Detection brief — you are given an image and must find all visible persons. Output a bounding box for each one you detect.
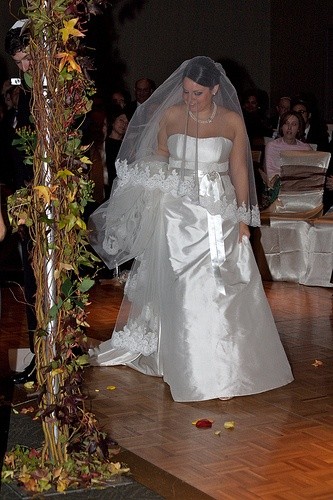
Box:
[241,92,333,214]
[0,33,94,385]
[86,56,296,403]
[0,78,155,192]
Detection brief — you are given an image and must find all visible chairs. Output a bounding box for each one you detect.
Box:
[261,136,333,289]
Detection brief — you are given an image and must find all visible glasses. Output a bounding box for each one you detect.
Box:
[291,99,304,104]
[298,110,310,114]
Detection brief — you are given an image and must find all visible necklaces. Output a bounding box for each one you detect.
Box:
[189,101,217,124]
